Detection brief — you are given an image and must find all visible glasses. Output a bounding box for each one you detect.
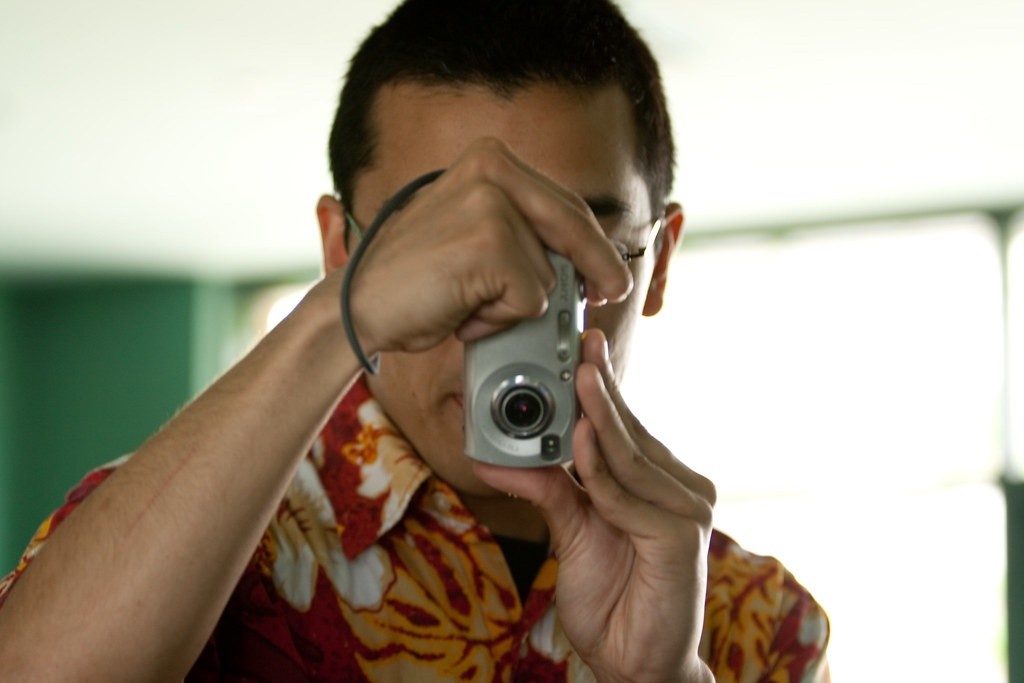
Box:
[340,208,664,265]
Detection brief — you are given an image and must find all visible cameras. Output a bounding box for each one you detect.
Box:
[464,254,589,471]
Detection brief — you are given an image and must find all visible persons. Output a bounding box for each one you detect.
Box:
[0,0,833,683]
